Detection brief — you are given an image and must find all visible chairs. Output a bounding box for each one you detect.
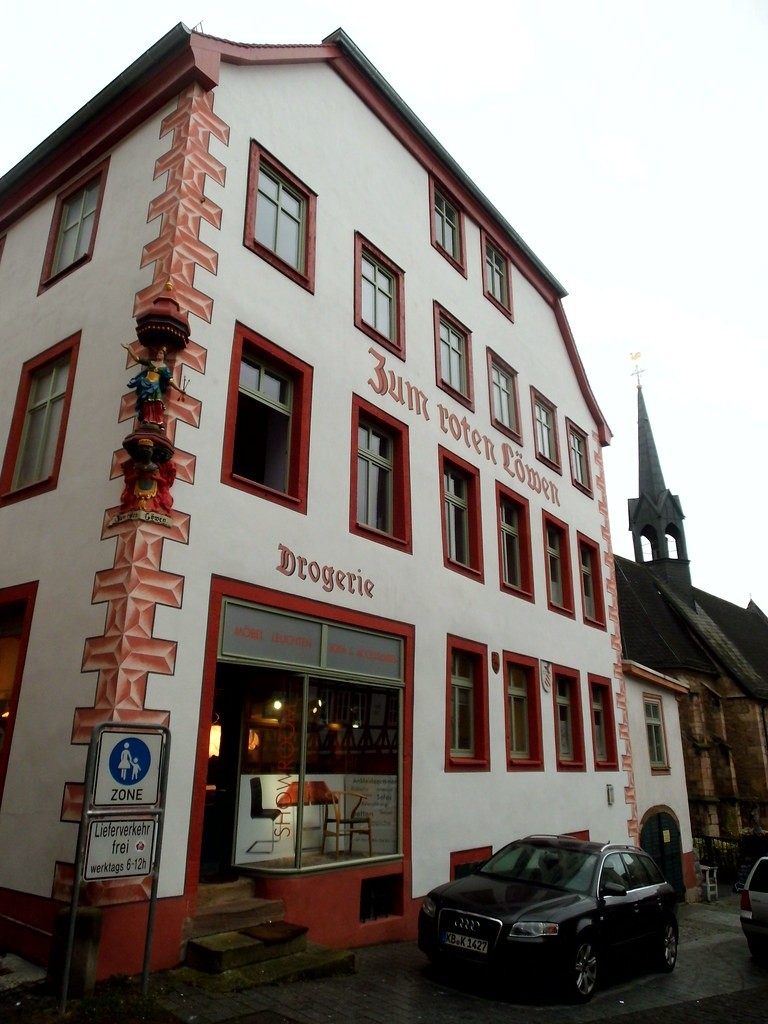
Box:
[321,790,371,861]
[529,852,560,885]
[245,777,281,853]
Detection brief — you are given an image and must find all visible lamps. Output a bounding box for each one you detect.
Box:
[606,784,615,806]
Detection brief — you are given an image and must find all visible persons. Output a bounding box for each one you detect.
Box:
[121,342,186,430]
[125,439,167,498]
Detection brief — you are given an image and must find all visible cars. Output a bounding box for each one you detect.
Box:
[739,854,768,962]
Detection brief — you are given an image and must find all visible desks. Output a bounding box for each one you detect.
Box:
[702,866,719,901]
[278,780,339,855]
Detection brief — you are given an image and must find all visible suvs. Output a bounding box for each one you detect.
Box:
[416,833,680,1005]
